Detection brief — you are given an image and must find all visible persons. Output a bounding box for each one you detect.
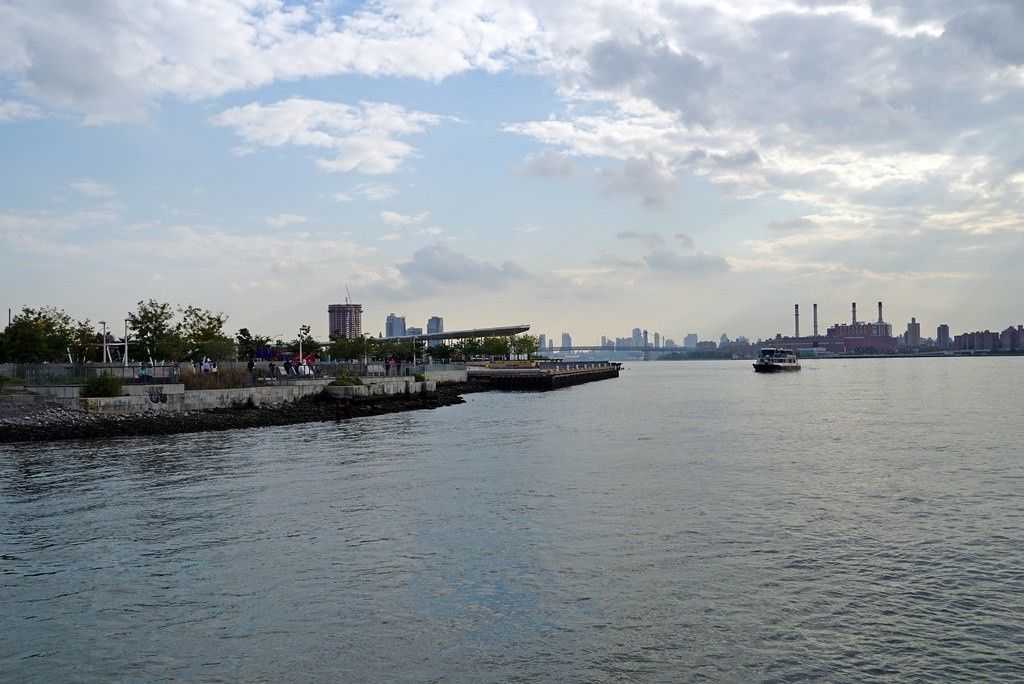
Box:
[385,355,401,376]
[138,366,148,378]
[247,359,319,379]
[203,360,217,373]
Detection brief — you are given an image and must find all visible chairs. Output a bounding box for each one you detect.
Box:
[368,364,386,377]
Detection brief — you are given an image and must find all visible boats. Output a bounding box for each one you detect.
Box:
[752,348,801,372]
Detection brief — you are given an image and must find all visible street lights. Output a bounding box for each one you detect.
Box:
[413,336,418,366]
[124,317,134,366]
[363,331,370,365]
[98,321,106,363]
[297,324,310,364]
